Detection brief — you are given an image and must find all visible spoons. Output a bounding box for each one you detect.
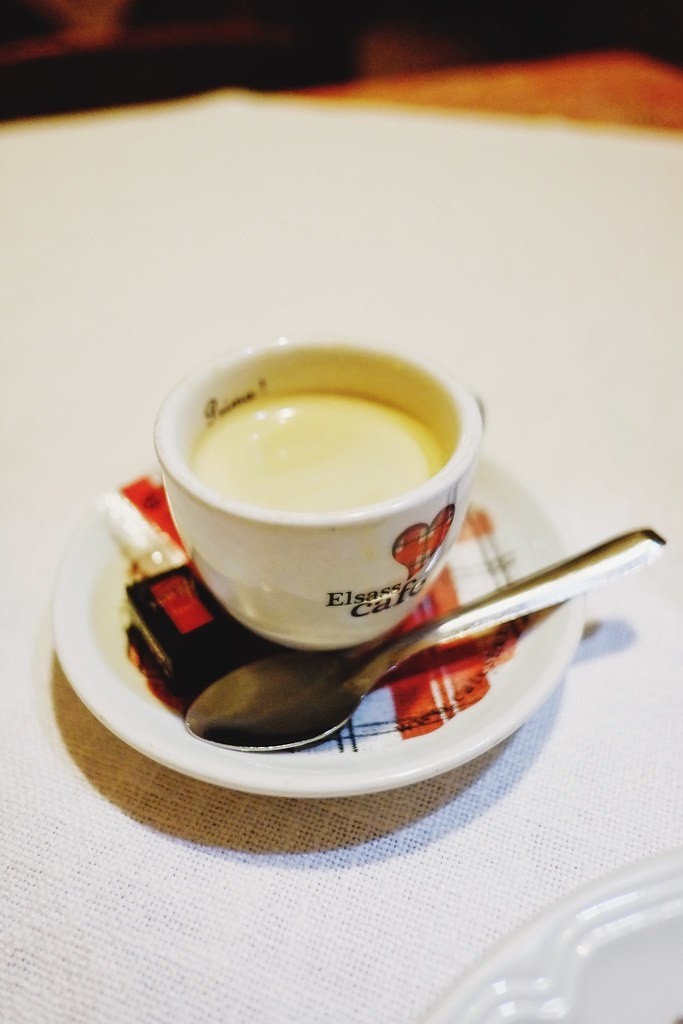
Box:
[184,527,665,746]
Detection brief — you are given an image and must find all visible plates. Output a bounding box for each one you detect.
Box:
[51,469,582,798]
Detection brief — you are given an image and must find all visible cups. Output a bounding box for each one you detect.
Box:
[152,337,484,650]
